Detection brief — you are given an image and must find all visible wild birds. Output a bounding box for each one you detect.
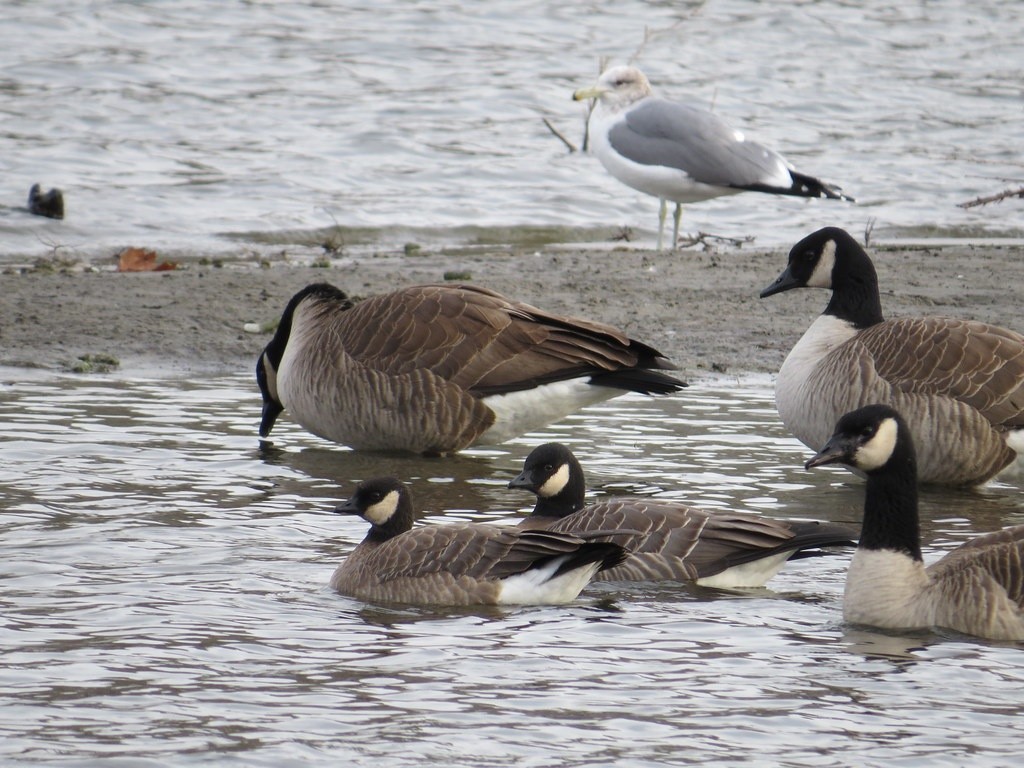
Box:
[255,283,689,455]
[805,403,1023,644]
[507,442,861,589]
[761,227,1024,492]
[572,66,855,252]
[328,476,651,607]
